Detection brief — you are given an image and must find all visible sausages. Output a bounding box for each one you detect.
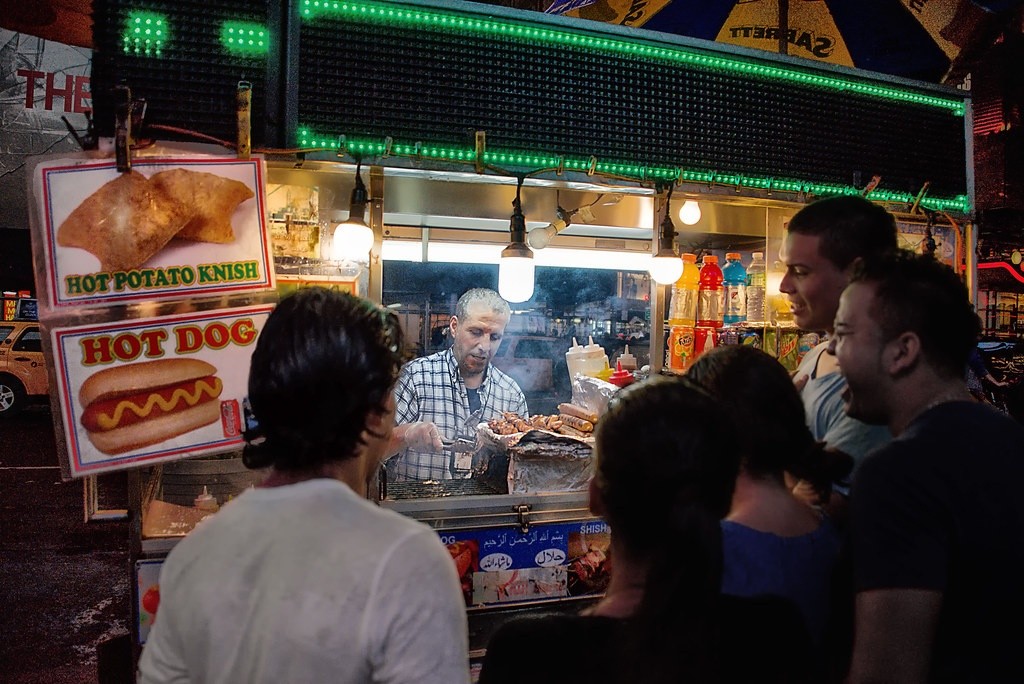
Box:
[488,404,599,437]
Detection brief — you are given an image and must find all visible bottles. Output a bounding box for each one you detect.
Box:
[597,361,615,382]
[616,344,638,373]
[583,335,610,380]
[565,337,585,386]
[194,486,219,513]
[720,253,748,323]
[696,256,724,327]
[667,253,700,327]
[609,361,634,387]
[745,252,766,324]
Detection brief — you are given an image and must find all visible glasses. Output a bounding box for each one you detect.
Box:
[608,374,717,414]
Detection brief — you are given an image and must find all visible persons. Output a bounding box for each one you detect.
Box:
[967,313,1009,402]
[138,286,472,684]
[826,247,1024,684]
[686,344,853,668]
[781,195,896,456]
[384,287,529,480]
[477,378,834,684]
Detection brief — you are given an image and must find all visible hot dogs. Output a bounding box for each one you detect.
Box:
[81,358,223,453]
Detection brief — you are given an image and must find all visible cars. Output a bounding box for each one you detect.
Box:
[614,326,645,341]
[488,336,560,392]
[0,321,56,413]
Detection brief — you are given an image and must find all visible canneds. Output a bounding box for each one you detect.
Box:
[671,324,820,374]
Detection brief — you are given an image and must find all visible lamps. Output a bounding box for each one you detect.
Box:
[332,162,375,259]
[498,178,535,304]
[529,190,606,249]
[649,182,684,286]
